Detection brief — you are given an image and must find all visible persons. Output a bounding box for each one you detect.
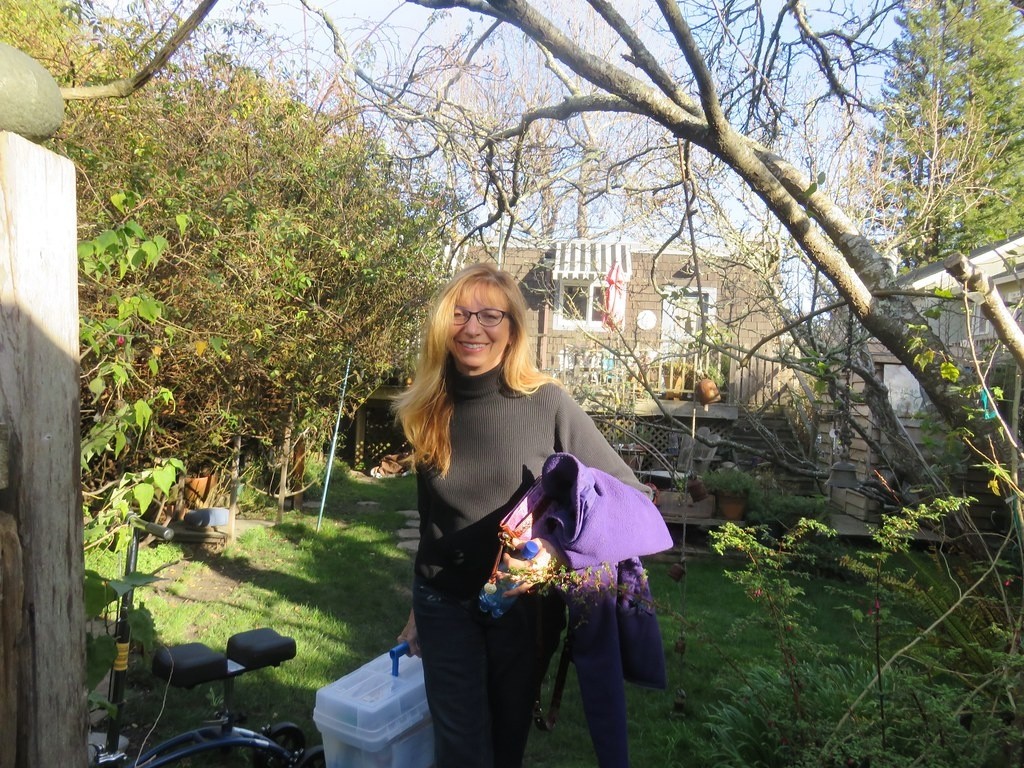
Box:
[391,263,652,767]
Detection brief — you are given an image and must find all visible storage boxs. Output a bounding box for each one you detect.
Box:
[312,641,436,768]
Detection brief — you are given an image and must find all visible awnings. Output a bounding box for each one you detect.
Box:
[550,241,632,282]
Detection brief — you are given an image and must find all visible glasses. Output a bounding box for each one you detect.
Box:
[453,307,516,327]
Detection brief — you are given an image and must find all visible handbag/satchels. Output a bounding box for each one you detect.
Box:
[485,474,577,595]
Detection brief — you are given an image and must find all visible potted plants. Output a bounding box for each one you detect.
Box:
[699,468,752,519]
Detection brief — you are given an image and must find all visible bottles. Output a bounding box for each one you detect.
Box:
[478,541,539,618]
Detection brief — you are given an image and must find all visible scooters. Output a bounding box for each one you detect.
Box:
[96,504,324,768]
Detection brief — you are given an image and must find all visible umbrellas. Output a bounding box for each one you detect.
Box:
[602,264,626,335]
